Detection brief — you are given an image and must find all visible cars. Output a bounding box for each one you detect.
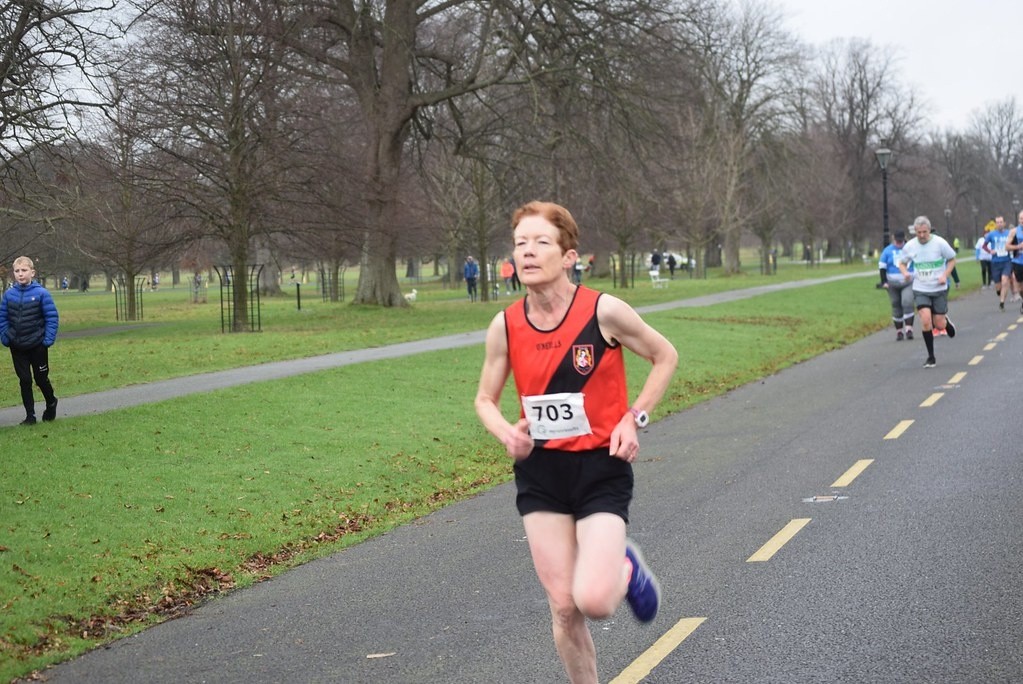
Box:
[646,253,696,270]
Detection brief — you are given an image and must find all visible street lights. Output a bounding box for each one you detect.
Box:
[1012,194,1019,226]
[875,136,891,247]
[972,207,979,243]
[944,205,951,243]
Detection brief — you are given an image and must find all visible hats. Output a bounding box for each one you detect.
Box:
[894,230,905,242]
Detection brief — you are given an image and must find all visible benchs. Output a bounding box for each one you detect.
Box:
[649,270,670,289]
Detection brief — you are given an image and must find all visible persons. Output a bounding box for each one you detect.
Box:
[288,267,295,285]
[574,255,594,282]
[501,254,521,295]
[0,256,59,425]
[667,253,675,276]
[143,273,158,292]
[474,200,678,684]
[195,271,200,292]
[974,210,1023,314]
[954,235,959,252]
[62,277,68,294]
[930,228,960,337]
[879,230,915,341]
[652,248,661,271]
[464,256,479,301]
[897,216,956,368]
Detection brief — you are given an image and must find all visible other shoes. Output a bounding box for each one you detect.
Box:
[1011,294,1022,303]
[997,290,1000,296]
[955,282,960,291]
[999,304,1005,312]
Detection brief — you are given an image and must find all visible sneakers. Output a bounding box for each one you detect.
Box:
[923,355,936,368]
[20,416,36,425]
[932,327,947,337]
[625,538,660,624]
[906,330,913,338]
[897,331,904,341]
[42,397,57,422]
[945,314,955,338]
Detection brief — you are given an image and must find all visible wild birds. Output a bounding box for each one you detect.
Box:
[405,289,417,302]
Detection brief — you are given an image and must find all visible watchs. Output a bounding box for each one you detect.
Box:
[628,408,650,427]
